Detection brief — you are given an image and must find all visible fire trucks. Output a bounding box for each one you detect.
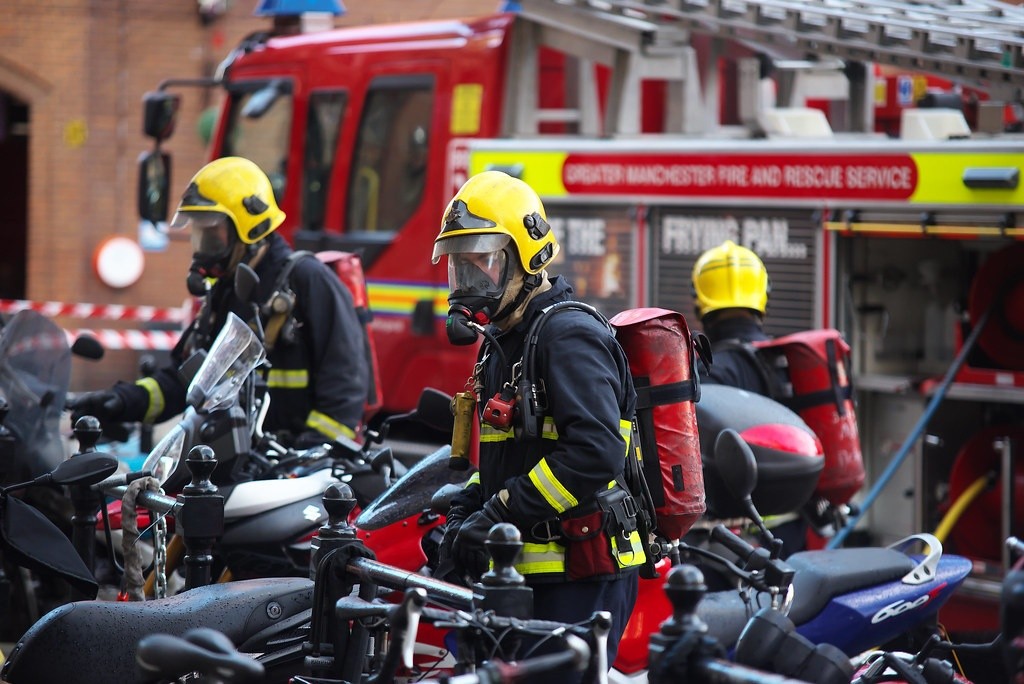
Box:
[136,0,1024,684]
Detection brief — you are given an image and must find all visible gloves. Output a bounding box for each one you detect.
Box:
[71,378,148,443]
[432,494,511,587]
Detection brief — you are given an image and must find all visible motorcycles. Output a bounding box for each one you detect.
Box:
[0,307,1024,684]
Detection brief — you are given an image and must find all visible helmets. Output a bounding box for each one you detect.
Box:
[177,157,286,243]
[691,239,767,321]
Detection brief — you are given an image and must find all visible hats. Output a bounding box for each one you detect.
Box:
[435,171,560,275]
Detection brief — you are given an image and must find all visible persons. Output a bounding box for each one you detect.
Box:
[69,156,372,454]
[692,240,794,409]
[431,169,651,684]
